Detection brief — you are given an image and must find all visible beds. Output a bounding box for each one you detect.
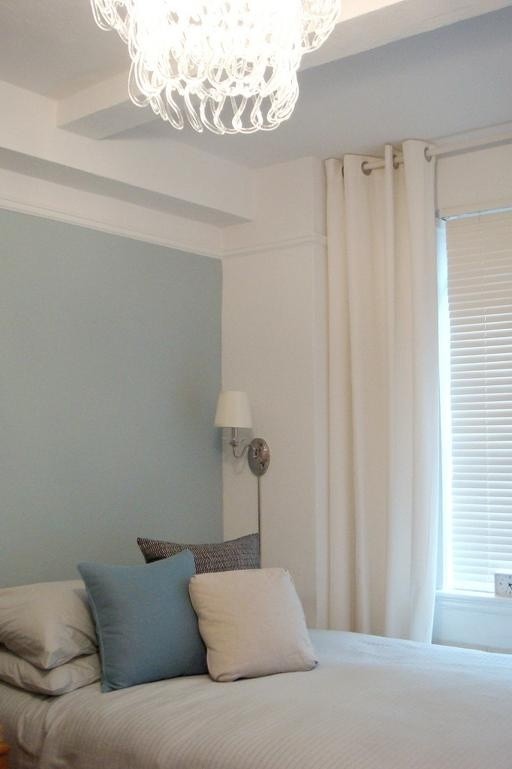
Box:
[1,630,512,769]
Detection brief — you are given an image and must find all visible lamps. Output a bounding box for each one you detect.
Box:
[88,1,343,134]
[214,390,268,479]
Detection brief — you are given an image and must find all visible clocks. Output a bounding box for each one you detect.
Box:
[495,573,512,597]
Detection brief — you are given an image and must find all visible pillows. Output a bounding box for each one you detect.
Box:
[73,549,208,694]
[190,566,318,684]
[2,578,101,670]
[1,645,101,697]
[137,534,263,573]
